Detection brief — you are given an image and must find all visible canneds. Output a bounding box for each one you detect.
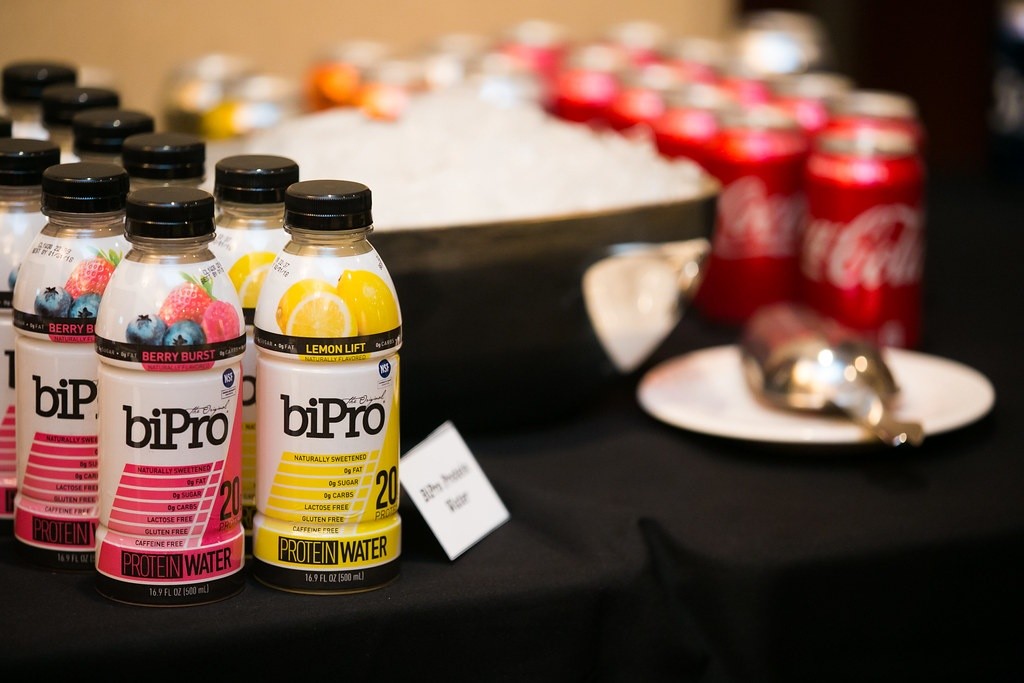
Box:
[304,19,929,347]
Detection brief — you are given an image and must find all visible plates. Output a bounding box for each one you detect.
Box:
[637,345,994,443]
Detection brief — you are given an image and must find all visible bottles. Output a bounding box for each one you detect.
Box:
[0,136,60,540]
[12,163,132,563]
[211,154,301,547]
[0,59,209,193]
[94,188,247,607]
[249,181,404,595]
